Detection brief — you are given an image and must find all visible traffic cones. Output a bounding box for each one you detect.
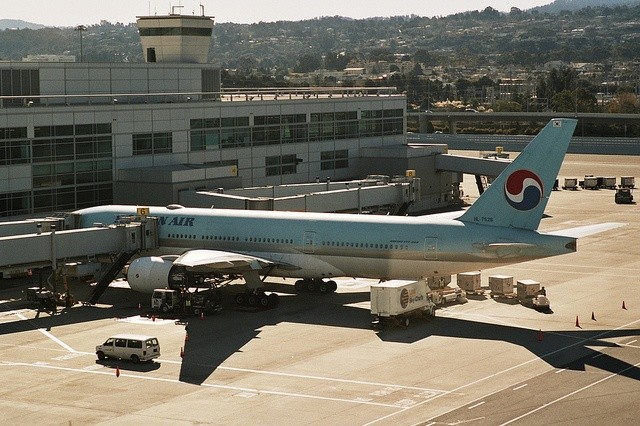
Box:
[184,331,189,342]
[576,316,579,326]
[198,309,205,321]
[592,312,594,319]
[622,300,625,309]
[116,367,119,377]
[537,329,544,341]
[180,347,184,357]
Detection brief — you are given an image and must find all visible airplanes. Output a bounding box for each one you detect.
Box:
[71,117,578,308]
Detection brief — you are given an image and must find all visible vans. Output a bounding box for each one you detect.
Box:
[95,335,161,364]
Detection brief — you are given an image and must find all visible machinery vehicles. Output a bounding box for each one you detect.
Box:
[614,189,634,204]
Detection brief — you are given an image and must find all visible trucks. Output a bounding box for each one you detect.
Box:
[427,272,551,312]
[150,286,214,316]
[369,279,437,327]
